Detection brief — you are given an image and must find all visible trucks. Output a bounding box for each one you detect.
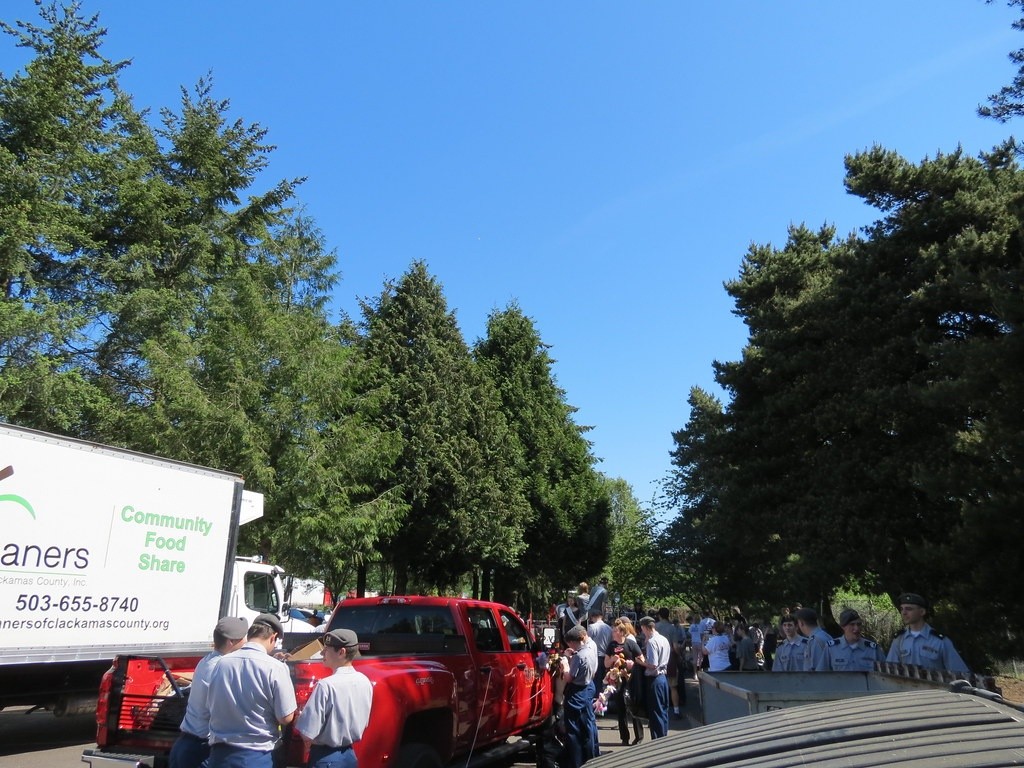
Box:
[0,419,317,718]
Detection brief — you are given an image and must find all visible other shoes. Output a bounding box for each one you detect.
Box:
[674,712,683,720]
[622,736,643,746]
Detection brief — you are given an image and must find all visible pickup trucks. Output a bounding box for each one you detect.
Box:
[84,592,557,768]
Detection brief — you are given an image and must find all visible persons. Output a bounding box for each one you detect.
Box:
[796,606,835,672]
[634,615,671,741]
[167,615,249,768]
[884,591,971,674]
[293,628,375,768]
[603,617,646,747]
[550,628,596,768]
[301,573,804,671]
[563,625,600,759]
[200,613,299,768]
[585,608,614,717]
[772,614,811,672]
[652,607,684,721]
[813,608,887,671]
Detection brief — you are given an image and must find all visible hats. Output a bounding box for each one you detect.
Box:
[620,616,630,623]
[796,607,817,622]
[322,629,359,649]
[779,614,798,625]
[839,610,861,625]
[254,612,286,640]
[897,593,927,607]
[562,628,581,640]
[657,607,669,619]
[638,616,654,625]
[588,609,603,617]
[216,614,250,641]
[570,624,586,633]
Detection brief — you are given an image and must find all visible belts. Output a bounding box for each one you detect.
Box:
[178,730,209,743]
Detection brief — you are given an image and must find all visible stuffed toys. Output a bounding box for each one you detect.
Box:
[592,653,631,717]
